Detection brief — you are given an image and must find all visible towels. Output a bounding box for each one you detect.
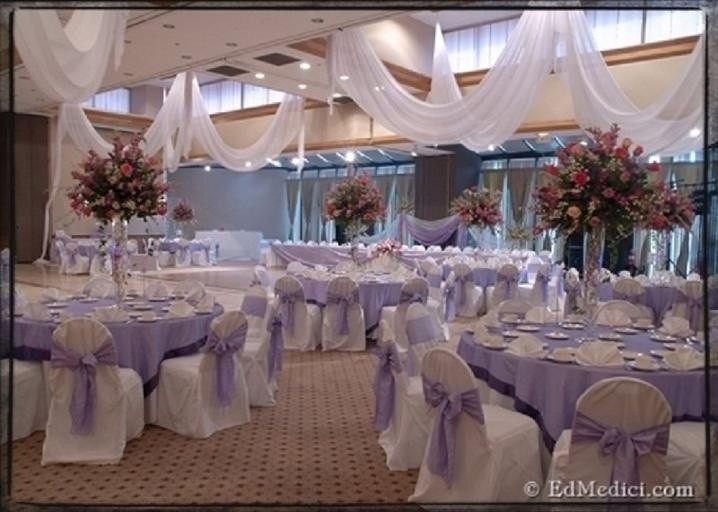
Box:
[168,302,194,318]
[576,342,625,368]
[525,307,555,323]
[482,310,502,328]
[37,288,67,302]
[663,318,691,337]
[665,348,701,371]
[89,283,115,299]
[184,289,203,302]
[24,303,53,323]
[196,294,215,310]
[507,336,548,358]
[599,311,632,328]
[92,308,129,323]
[473,323,489,346]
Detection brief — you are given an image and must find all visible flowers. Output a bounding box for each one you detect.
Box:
[452,186,504,224]
[62,131,170,221]
[174,198,191,220]
[323,172,388,223]
[533,124,696,236]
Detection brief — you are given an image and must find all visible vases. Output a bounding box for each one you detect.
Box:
[350,216,360,260]
[583,228,606,291]
[111,216,131,280]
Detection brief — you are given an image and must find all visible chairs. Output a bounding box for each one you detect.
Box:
[671,421,708,500]
[158,310,251,440]
[39,318,144,460]
[521,264,552,301]
[668,280,704,332]
[375,319,426,472]
[544,377,672,501]
[404,302,438,373]
[273,276,321,351]
[241,299,285,408]
[429,271,456,342]
[454,264,485,317]
[613,279,646,306]
[138,236,220,267]
[267,237,378,261]
[492,264,521,303]
[393,228,544,265]
[410,348,552,503]
[378,277,429,343]
[49,230,107,275]
[320,276,367,351]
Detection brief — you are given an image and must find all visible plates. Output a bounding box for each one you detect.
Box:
[632,323,655,329]
[599,334,623,339]
[467,326,488,333]
[483,321,500,328]
[650,335,676,341]
[610,342,624,347]
[622,351,639,359]
[614,327,638,333]
[628,360,660,371]
[14,291,214,322]
[548,353,573,362]
[650,349,673,357]
[482,341,508,348]
[564,324,583,329]
[545,332,570,339]
[664,343,684,350]
[516,326,539,331]
[502,331,519,337]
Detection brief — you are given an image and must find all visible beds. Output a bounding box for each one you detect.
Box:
[13,290,223,384]
